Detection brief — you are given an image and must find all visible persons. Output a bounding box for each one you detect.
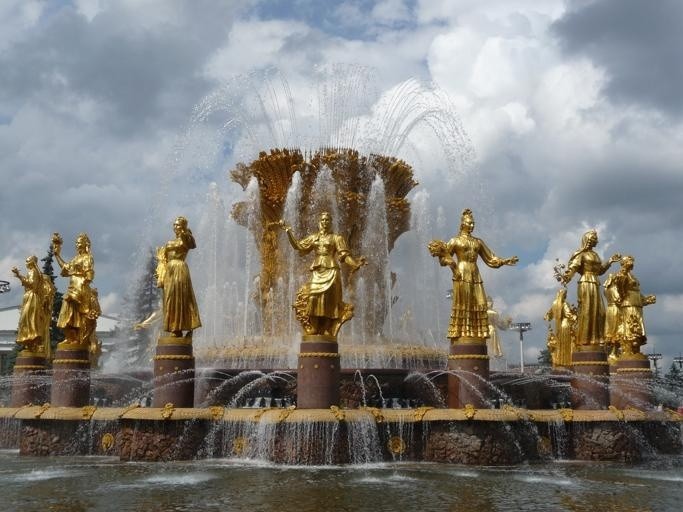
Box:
[49,231,95,346]
[10,254,47,353]
[275,211,369,336]
[543,289,578,367]
[88,286,102,354]
[484,296,514,358]
[553,228,624,347]
[603,273,623,346]
[428,209,519,339]
[151,216,202,338]
[617,252,657,352]
[39,273,54,359]
[132,300,165,348]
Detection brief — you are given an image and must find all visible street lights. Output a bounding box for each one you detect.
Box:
[0,280,10,294]
[648,353,663,379]
[508,323,531,375]
[672,357,683,371]
